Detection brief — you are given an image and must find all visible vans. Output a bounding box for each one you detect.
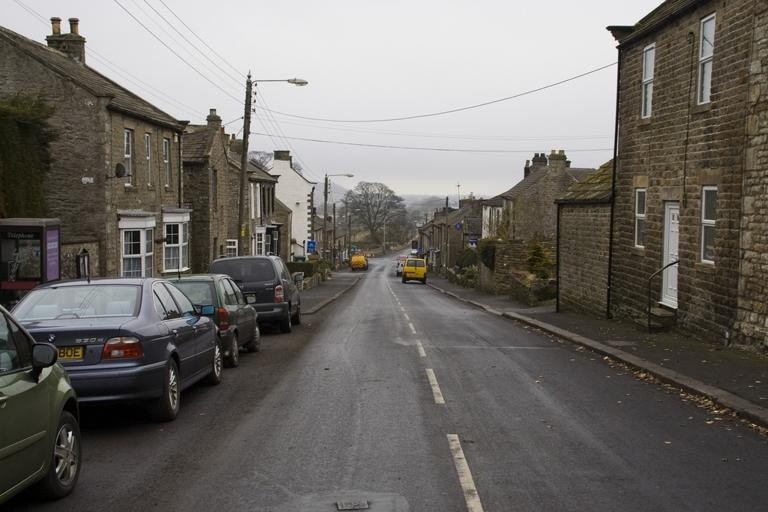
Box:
[402,258,427,284]
[352,256,368,270]
[209,255,299,335]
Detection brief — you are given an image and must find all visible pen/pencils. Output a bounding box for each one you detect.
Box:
[402,258,427,284]
[352,256,368,270]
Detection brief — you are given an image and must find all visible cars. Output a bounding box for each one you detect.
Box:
[0,304,83,505]
[8,278,224,422]
[162,273,260,368]
[396,262,403,276]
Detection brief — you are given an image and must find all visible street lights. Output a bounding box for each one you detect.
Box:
[323,173,354,261]
[349,208,360,265]
[238,69,308,256]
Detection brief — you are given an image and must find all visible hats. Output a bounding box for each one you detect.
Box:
[396,262,403,276]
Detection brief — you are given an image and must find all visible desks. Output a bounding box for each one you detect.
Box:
[238,69,308,256]
[323,173,354,261]
[349,208,360,265]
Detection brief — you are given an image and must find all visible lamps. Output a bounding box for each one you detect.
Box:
[31,301,130,318]
